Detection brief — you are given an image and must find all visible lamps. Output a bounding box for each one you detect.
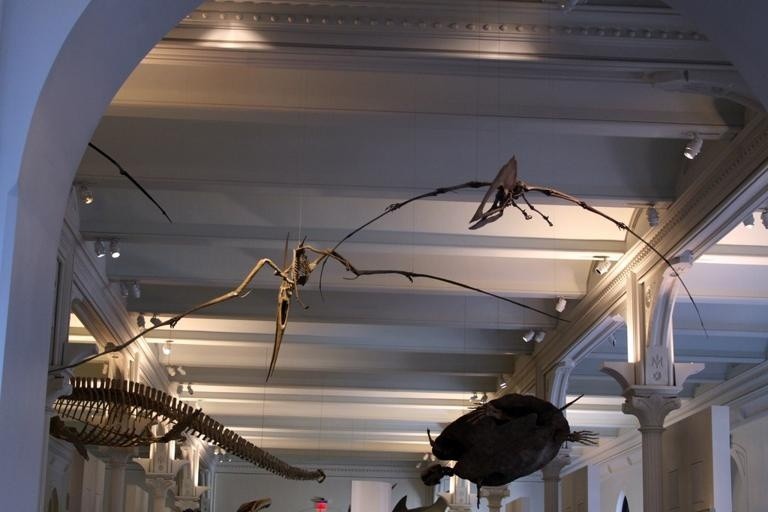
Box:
[645,204,662,229]
[593,256,612,276]
[682,135,704,162]
[469,371,512,403]
[555,296,567,315]
[522,329,546,344]
[75,182,194,396]
